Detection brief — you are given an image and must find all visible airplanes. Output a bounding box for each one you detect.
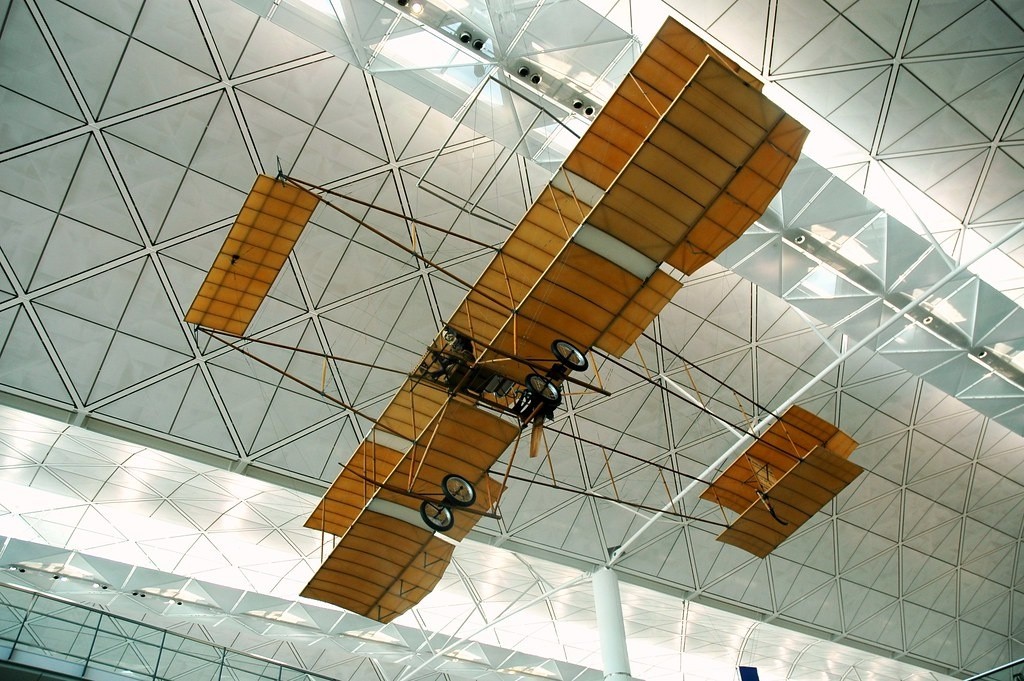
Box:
[181,14,866,625]
